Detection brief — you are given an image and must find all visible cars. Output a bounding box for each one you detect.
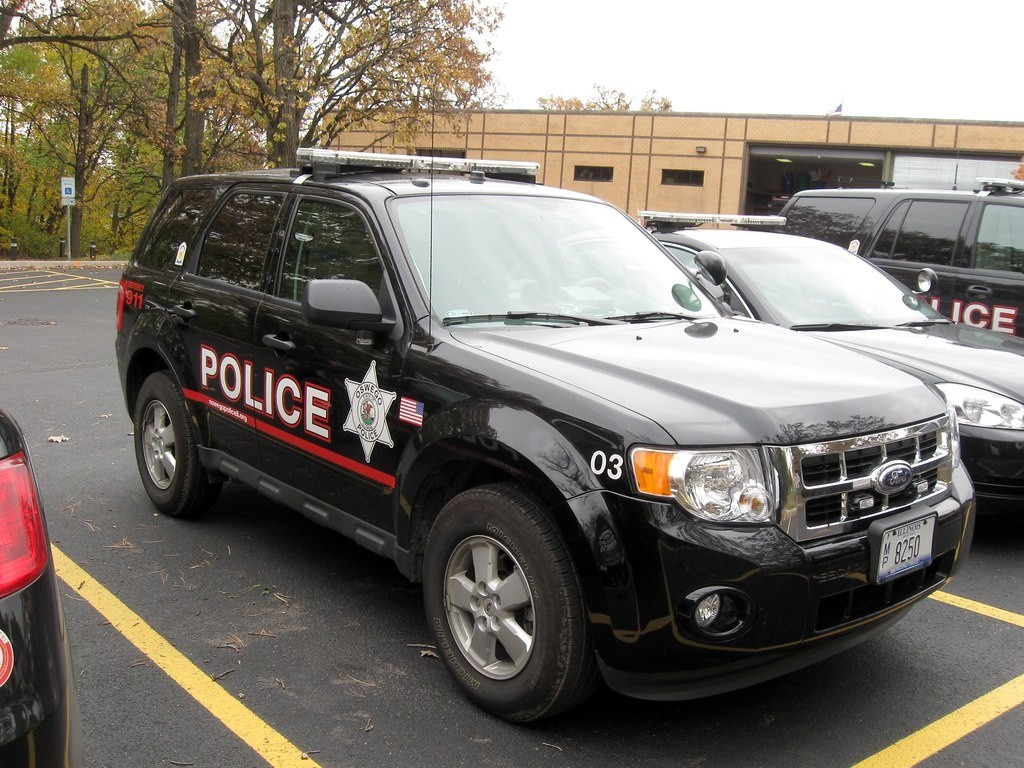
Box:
[556,207,1024,526]
[0,411,72,768]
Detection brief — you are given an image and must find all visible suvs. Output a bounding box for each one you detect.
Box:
[775,175,1023,343]
[115,149,976,726]
